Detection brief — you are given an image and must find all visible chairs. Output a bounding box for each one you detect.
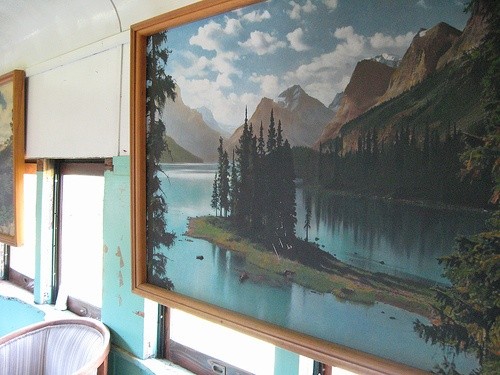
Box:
[0,318,112,375]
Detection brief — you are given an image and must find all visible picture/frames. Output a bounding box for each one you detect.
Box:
[0,69,26,247]
[129,0,500,375]
